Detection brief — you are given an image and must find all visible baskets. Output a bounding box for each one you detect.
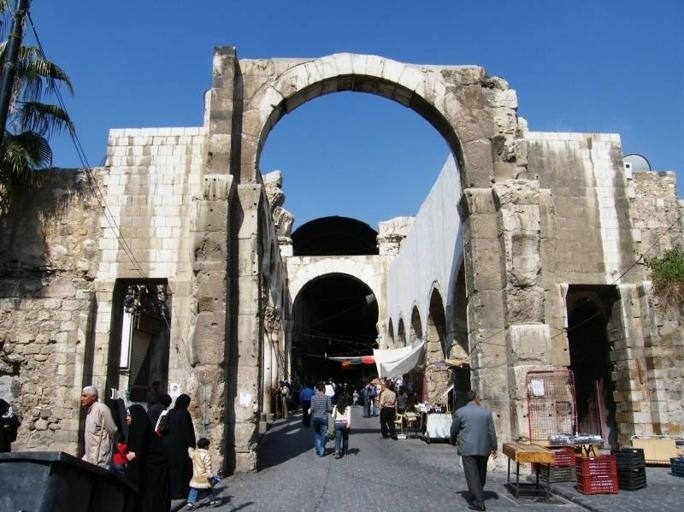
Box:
[539,446,578,467]
[609,446,647,469]
[669,456,684,477]
[575,454,617,477]
[575,475,618,494]
[616,467,646,490]
[538,464,577,483]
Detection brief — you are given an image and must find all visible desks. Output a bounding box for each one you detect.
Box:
[502,442,555,500]
[532,440,605,457]
[421,412,453,445]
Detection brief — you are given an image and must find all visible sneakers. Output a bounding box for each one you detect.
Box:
[314,446,350,460]
[186,499,224,510]
[467,502,485,511]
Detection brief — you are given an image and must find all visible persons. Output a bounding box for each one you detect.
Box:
[80,380,222,511]
[278,373,420,459]
[449,390,498,511]
